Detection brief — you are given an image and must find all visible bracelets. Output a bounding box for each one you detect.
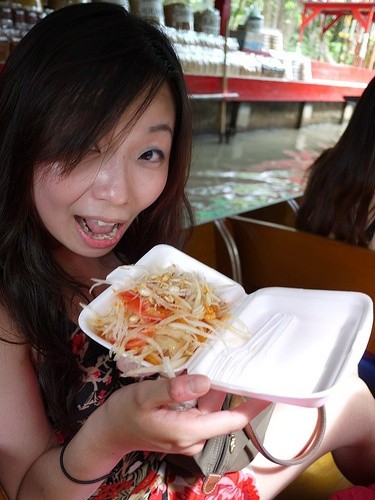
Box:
[59,441,124,484]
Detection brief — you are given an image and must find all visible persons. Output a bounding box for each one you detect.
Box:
[295,72,374,255]
[0,2,375,500]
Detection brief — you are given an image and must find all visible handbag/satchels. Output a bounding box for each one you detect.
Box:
[153,371,327,481]
